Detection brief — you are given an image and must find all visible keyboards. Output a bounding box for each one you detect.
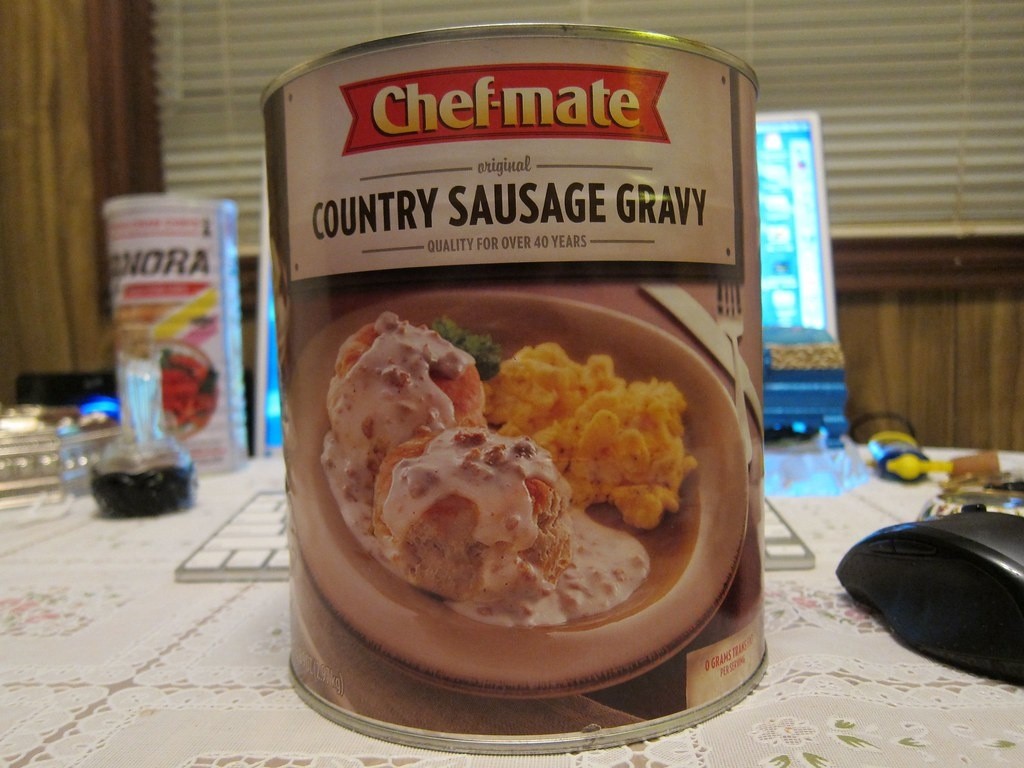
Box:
[172,488,813,586]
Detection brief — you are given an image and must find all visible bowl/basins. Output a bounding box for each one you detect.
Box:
[285,288,751,701]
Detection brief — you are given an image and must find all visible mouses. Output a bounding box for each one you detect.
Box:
[836,501,1022,688]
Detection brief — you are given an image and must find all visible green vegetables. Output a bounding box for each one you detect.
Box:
[429,315,501,381]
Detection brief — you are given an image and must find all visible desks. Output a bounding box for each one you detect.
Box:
[0,439,1024,768]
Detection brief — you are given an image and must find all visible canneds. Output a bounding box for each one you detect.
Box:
[103,193,248,473]
[257,17,769,756]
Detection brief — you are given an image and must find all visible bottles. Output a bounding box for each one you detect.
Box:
[87,345,195,519]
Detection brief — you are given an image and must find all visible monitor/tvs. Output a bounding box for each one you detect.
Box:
[755,112,851,440]
